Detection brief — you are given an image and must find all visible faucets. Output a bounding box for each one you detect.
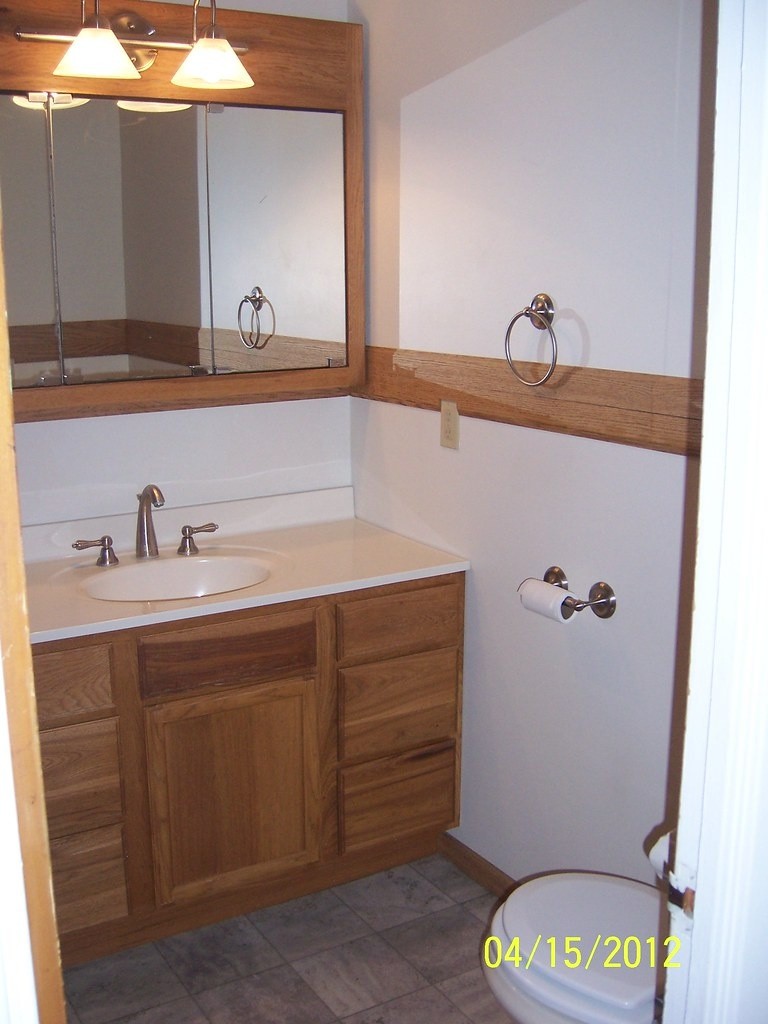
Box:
[136,484,165,559]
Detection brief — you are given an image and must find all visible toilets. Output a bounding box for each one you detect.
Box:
[479,829,676,1024]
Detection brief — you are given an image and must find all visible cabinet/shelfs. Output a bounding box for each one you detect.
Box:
[30,570,466,968]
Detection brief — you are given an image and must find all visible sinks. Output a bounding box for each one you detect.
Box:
[83,557,270,600]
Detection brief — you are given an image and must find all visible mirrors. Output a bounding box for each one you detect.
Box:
[0,87,347,390]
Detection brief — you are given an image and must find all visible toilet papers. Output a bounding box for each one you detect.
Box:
[516,577,578,624]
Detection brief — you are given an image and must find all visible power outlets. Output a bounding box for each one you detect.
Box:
[440,398,459,450]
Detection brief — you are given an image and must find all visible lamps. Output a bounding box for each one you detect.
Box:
[16,1,257,90]
